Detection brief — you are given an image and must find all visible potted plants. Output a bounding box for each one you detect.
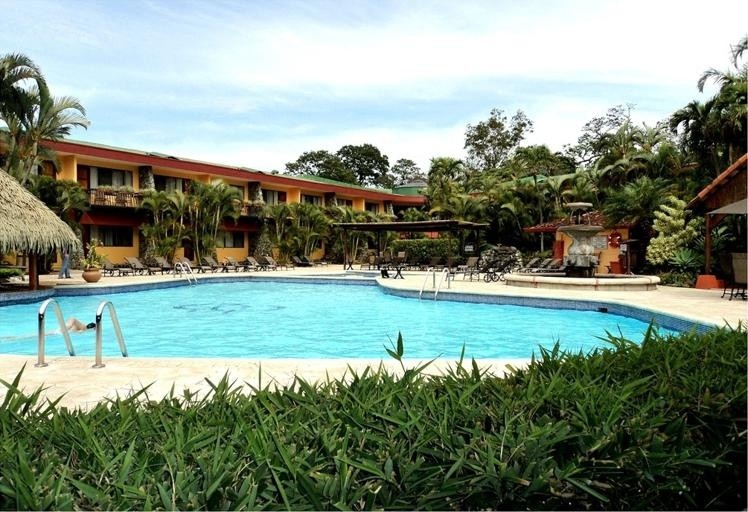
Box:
[81,241,102,282]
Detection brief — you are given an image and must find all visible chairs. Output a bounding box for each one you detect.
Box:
[377,257,563,282]
[102,255,294,277]
[722,264,744,302]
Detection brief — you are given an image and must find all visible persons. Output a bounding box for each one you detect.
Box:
[57,244,73,279]
